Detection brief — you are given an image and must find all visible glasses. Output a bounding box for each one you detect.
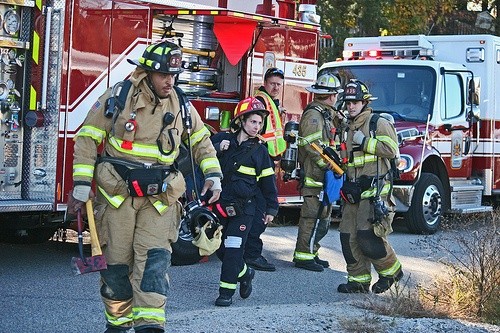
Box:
[270,68,284,75]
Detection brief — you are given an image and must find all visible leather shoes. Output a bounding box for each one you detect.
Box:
[246,256,275,271]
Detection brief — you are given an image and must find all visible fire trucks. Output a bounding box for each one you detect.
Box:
[0,0,332,265]
[317,35,500,235]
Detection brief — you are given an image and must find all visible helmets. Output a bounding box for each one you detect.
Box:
[230,97,270,127]
[339,79,378,101]
[305,72,344,94]
[127,40,184,74]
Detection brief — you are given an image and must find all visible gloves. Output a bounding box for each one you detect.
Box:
[353,131,365,145]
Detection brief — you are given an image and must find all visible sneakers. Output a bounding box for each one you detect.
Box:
[314,256,328,268]
[295,260,324,271]
[240,268,255,298]
[372,271,403,293]
[338,282,370,293]
[215,293,233,306]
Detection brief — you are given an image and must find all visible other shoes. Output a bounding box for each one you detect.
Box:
[104,328,131,333]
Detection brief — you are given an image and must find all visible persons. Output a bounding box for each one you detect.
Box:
[244,68,286,272]
[292,73,344,271]
[71,43,224,333]
[325,79,404,294]
[211,97,279,307]
